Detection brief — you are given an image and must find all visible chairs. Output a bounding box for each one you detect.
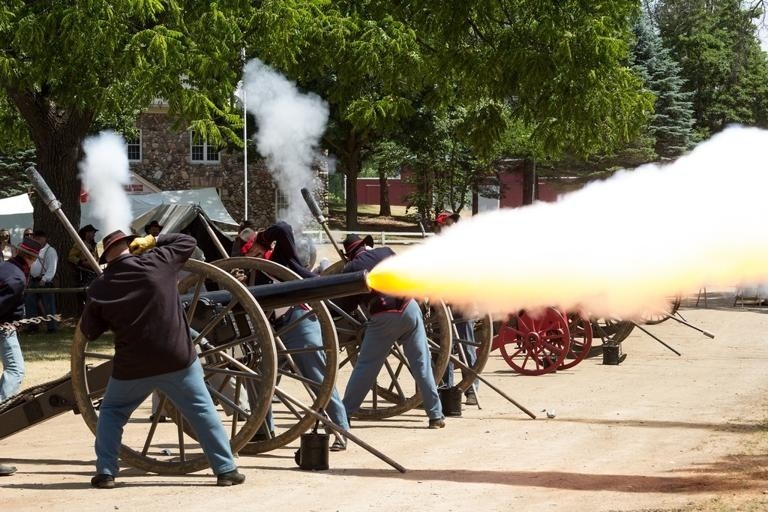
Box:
[150,396,282,442]
[217,470,246,487]
[330,434,347,452]
[91,474,114,489]
[429,419,445,430]
[0,462,18,476]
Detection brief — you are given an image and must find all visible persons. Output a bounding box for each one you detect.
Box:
[330,233,444,429]
[433,212,479,404]
[231,220,350,452]
[145,220,164,236]
[0,224,101,333]
[0,237,44,475]
[80,231,245,488]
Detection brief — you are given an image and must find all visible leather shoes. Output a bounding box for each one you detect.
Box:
[129,235,156,257]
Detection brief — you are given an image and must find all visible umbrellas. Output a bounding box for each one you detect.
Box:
[466,395,477,406]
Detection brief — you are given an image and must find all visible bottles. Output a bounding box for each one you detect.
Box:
[144,220,164,236]
[435,210,461,227]
[239,227,266,254]
[99,229,140,264]
[341,234,375,256]
[13,239,42,262]
[77,224,99,237]
